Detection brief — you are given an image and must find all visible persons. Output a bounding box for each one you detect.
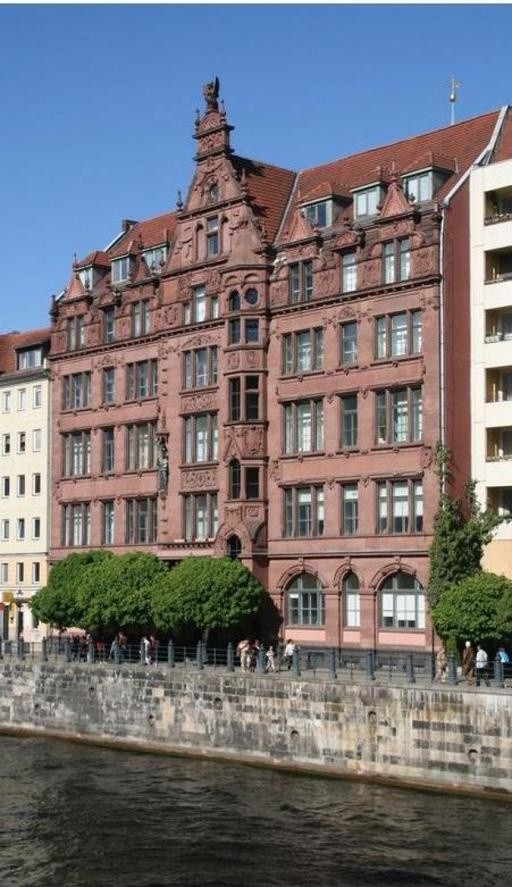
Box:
[495,648,510,683]
[237,637,252,671]
[250,637,261,672]
[265,644,275,671]
[433,647,448,683]
[474,644,489,686]
[282,638,299,669]
[462,639,475,686]
[66,630,157,663]
